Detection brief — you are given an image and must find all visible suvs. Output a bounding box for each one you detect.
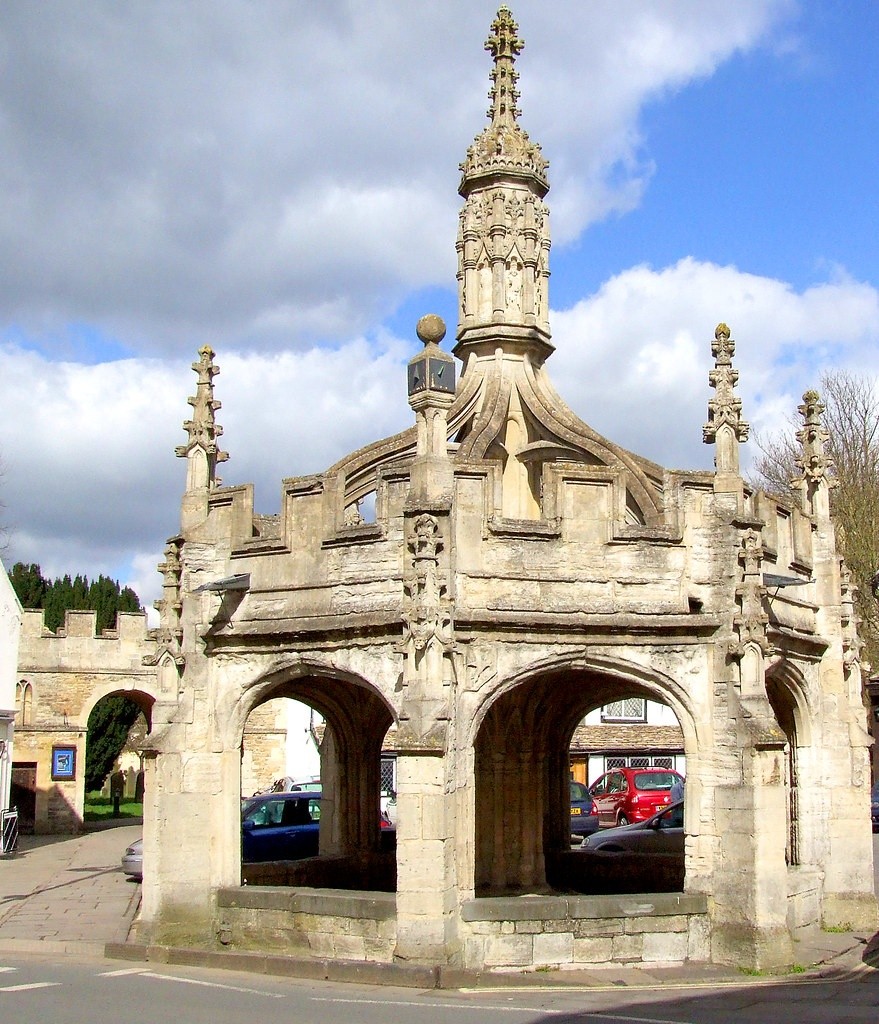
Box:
[589,766,686,829]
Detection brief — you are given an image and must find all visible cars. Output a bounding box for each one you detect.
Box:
[242,780,397,863]
[122,838,142,877]
[569,781,599,837]
[871,780,879,834]
[580,800,684,853]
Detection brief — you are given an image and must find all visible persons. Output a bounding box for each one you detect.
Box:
[670,777,686,814]
[623,778,627,787]
[386,791,397,824]
